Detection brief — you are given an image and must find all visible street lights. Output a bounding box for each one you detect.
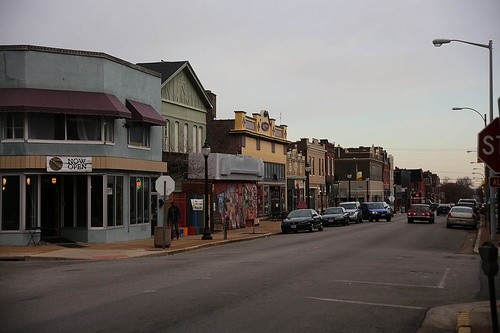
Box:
[305,163,311,208]
[392,183,397,213]
[451,106,487,129]
[432,39,495,240]
[201,142,213,240]
[365,176,369,202]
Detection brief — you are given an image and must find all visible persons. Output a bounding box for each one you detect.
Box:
[167,201,181,240]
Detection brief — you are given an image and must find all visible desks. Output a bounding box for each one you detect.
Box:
[27,226,50,248]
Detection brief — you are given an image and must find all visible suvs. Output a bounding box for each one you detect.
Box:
[338,200,363,223]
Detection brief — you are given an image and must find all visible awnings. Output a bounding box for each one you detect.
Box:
[0,87,131,119]
[125,99,167,126]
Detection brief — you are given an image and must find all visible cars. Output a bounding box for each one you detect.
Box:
[281,209,323,232]
[361,201,393,222]
[446,198,482,229]
[322,206,350,226]
[431,203,456,216]
[407,203,434,224]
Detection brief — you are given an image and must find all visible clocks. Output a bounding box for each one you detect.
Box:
[262,122,269,131]
